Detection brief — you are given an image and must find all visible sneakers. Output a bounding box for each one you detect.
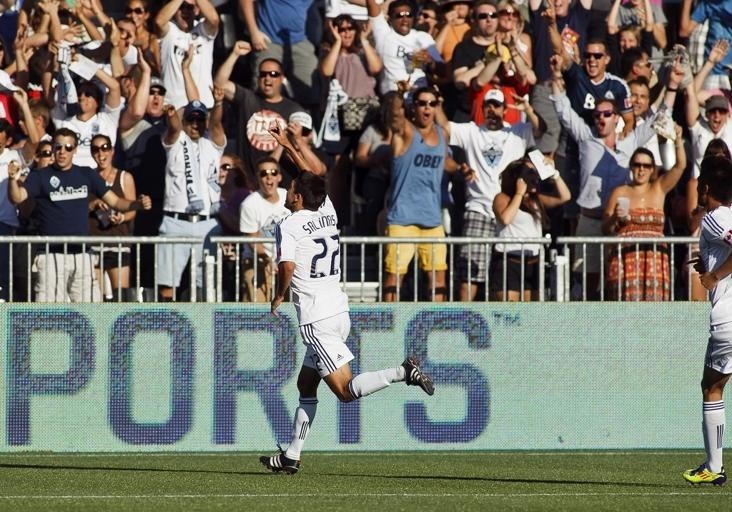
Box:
[402,357,434,395]
[683,463,726,487]
[260,443,299,474]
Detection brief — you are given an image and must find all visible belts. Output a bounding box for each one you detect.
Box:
[509,257,538,265]
[165,211,221,221]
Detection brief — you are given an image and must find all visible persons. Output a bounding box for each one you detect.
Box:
[1,2,732,299]
[259,119,434,473]
[681,153,732,488]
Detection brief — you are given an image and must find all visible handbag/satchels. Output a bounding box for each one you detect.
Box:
[343,95,379,129]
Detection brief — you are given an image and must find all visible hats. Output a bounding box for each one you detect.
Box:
[704,95,729,114]
[484,90,504,102]
[184,101,206,114]
[150,77,165,86]
[289,112,312,130]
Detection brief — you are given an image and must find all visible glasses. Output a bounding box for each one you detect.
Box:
[261,169,278,177]
[339,26,355,33]
[418,100,437,108]
[640,62,652,68]
[633,162,653,170]
[393,11,412,19]
[418,11,436,21]
[257,70,279,78]
[91,144,109,153]
[593,111,613,118]
[222,164,231,169]
[126,7,143,15]
[54,143,72,151]
[478,13,498,19]
[583,52,602,59]
[500,10,518,18]
[150,89,166,95]
[43,150,52,156]
[184,114,203,123]
